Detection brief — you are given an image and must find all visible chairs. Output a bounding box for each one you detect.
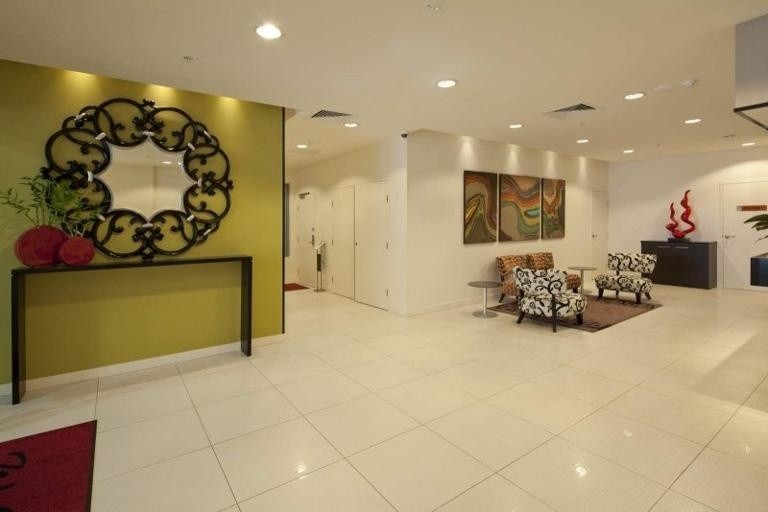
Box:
[495,255,530,303]
[529,252,582,293]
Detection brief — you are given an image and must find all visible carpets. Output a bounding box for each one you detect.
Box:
[284,281,310,292]
[486,293,664,334]
[0,417,99,512]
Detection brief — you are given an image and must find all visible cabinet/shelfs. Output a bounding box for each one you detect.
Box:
[640,240,718,290]
[750,251,768,287]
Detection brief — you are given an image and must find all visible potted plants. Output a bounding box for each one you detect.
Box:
[1,171,81,269]
[49,193,108,267]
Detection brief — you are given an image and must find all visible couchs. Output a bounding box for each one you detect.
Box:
[594,252,658,305]
[511,266,588,333]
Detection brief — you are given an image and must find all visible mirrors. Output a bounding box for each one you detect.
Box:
[35,96,236,264]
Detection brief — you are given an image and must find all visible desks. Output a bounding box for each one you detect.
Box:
[11,253,255,404]
[568,265,599,293]
[467,280,504,320]
